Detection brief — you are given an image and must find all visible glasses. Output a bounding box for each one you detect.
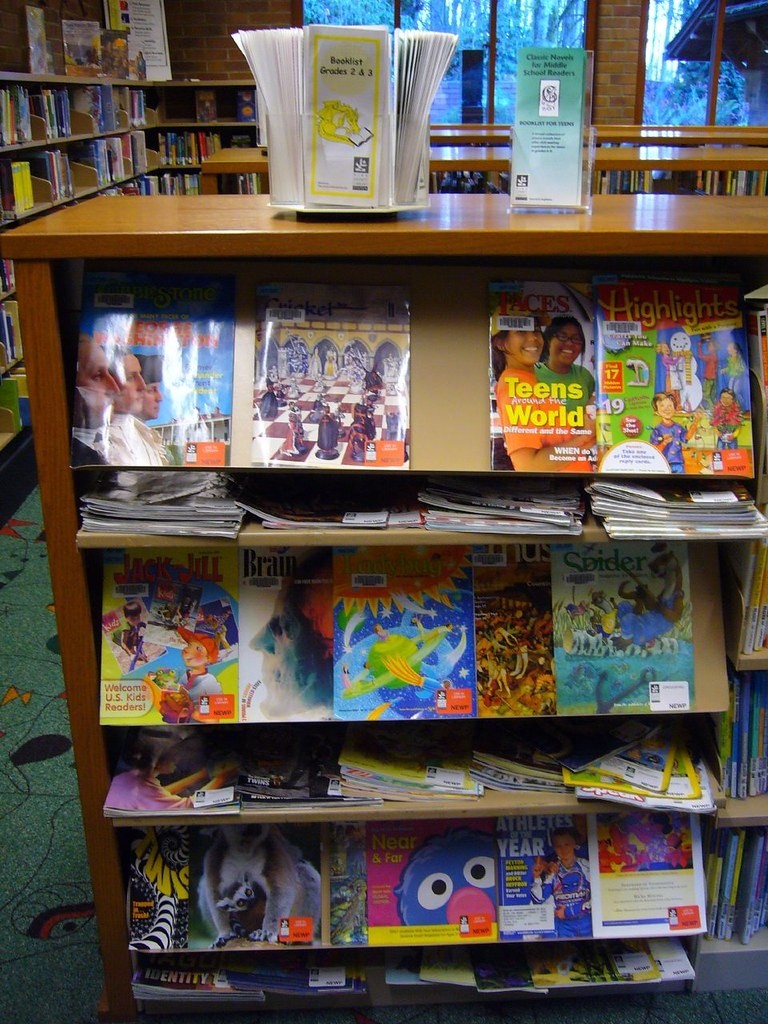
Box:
[548,332,585,345]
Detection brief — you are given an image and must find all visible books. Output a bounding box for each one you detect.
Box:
[488,269,755,479]
[704,303,768,945]
[0,259,32,432]
[248,284,410,470]
[79,476,768,1002]
[69,258,238,470]
[0,5,261,220]
[597,169,767,196]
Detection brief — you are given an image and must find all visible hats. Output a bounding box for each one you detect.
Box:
[134,354,163,384]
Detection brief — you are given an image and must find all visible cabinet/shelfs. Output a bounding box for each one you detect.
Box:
[0,71,768,1024]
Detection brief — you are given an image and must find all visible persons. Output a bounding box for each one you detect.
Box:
[528,814,593,937]
[495,316,596,472]
[74,334,170,467]
